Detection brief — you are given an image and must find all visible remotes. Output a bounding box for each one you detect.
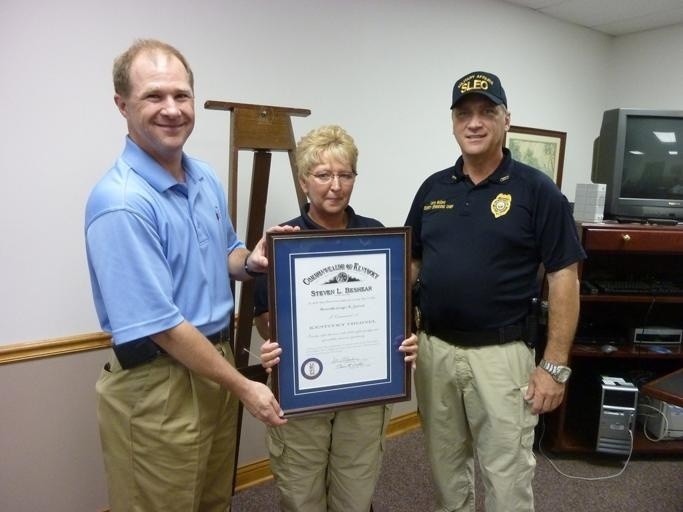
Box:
[647,218,678,225]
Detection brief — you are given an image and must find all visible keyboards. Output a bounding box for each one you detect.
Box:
[597,280,683,296]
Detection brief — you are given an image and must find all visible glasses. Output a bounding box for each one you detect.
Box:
[307,169,355,185]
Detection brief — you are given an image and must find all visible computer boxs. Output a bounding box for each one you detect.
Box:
[594,374,639,456]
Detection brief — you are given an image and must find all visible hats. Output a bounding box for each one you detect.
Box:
[449,70,508,112]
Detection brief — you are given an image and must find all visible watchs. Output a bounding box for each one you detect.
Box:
[539,357,574,385]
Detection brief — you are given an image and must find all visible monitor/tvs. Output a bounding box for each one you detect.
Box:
[591,108,683,222]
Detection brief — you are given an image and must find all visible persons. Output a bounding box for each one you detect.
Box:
[84,35,302,512]
[402,70,588,511]
[252,124,420,511]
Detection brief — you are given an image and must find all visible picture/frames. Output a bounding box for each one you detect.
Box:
[502,125,567,191]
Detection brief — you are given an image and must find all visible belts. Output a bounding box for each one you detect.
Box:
[419,319,524,349]
[204,324,230,346]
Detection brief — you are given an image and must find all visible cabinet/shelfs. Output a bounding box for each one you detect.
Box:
[534,222,683,453]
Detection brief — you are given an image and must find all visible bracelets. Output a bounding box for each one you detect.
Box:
[243,250,265,278]
